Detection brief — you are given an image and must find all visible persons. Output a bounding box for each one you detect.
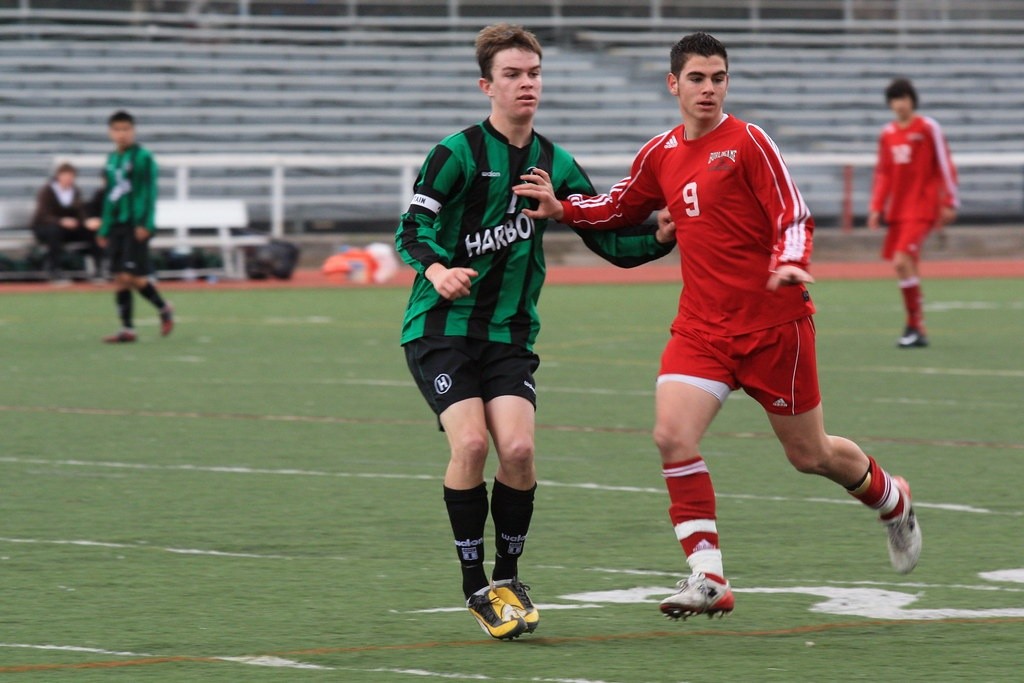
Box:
[32,164,111,278]
[96,111,175,342]
[868,79,960,346]
[510,34,922,618]
[395,23,677,639]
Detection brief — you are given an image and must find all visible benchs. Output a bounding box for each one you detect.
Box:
[2,195,269,281]
[0,0,1024,229]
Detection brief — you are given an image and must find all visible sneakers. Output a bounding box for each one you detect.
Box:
[659,571,735,618]
[465,583,530,641]
[160,303,173,335]
[877,474,923,575]
[103,329,137,341]
[899,329,927,346]
[489,571,541,632]
